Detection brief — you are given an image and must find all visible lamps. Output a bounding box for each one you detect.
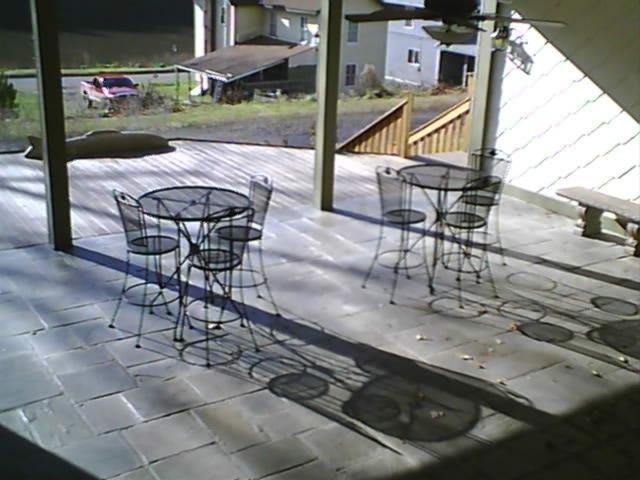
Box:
[422,19,479,47]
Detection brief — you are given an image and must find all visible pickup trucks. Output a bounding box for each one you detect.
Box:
[80,74,144,109]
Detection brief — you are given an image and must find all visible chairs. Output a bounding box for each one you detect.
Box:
[108,173,284,374]
[361,146,512,316]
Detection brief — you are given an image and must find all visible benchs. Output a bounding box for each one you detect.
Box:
[554,188,640,257]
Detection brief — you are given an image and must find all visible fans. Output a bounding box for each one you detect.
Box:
[341,1,571,29]
